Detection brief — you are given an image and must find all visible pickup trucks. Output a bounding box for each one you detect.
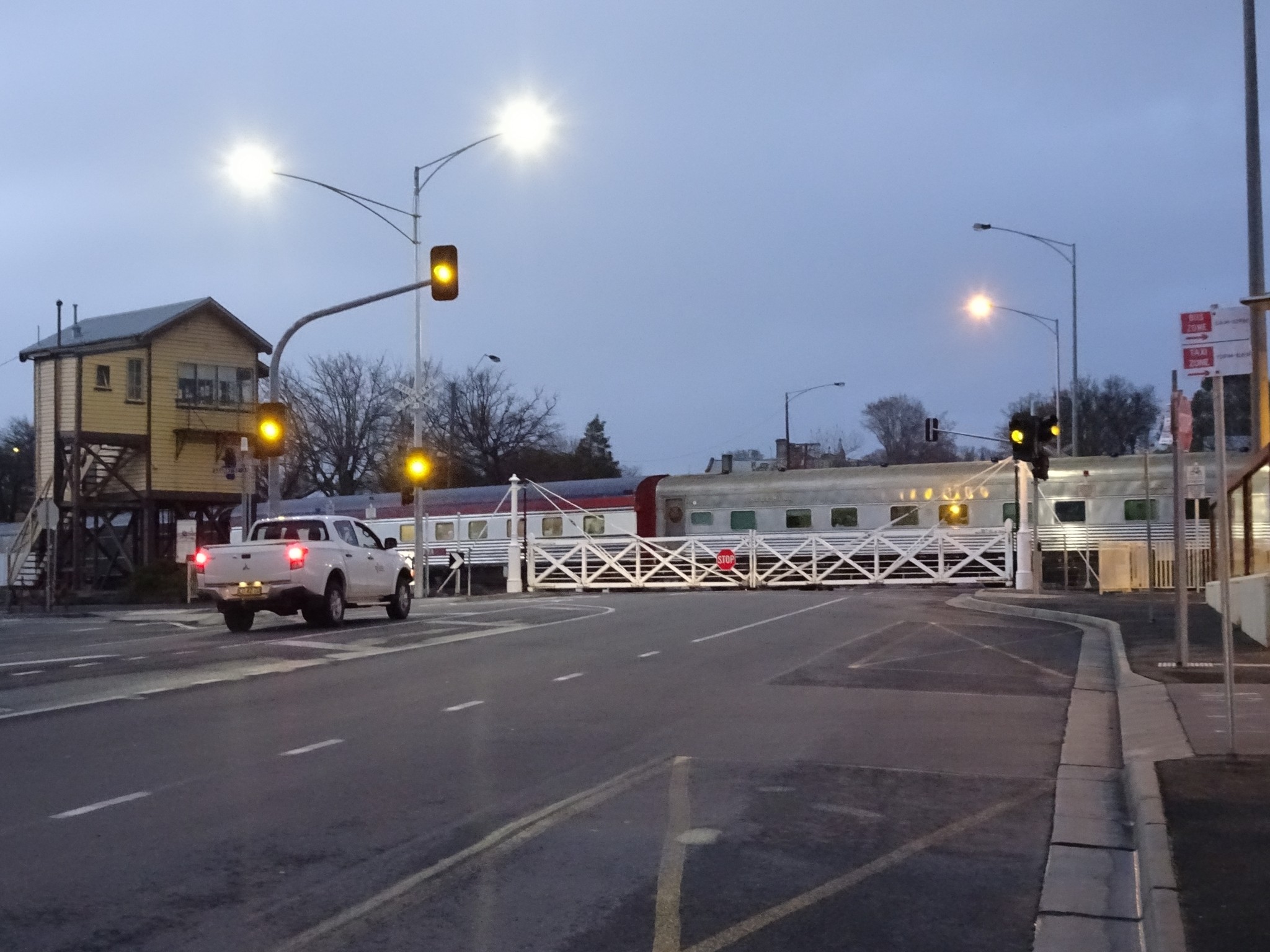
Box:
[194,513,415,630]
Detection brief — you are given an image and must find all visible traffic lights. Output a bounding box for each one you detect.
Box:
[254,401,286,458]
[400,473,414,507]
[406,446,433,482]
[1038,415,1058,442]
[1008,414,1034,464]
[427,245,458,302]
[1032,458,1048,481]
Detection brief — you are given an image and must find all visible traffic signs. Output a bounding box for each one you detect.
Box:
[1179,310,1254,381]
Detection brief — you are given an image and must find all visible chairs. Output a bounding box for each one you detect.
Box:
[308,527,321,540]
[284,528,300,540]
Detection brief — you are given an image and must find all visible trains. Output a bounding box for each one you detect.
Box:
[230,449,1269,585]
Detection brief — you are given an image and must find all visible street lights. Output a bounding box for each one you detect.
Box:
[969,297,1062,455]
[233,112,543,600]
[973,222,1078,459]
[447,353,500,487]
[783,381,846,467]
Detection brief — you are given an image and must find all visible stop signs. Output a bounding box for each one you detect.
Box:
[715,548,736,572]
